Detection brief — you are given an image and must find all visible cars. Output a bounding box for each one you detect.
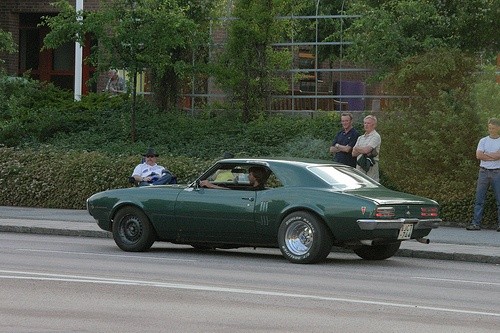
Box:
[86,159,442,265]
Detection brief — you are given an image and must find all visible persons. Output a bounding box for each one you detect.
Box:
[352,115,381,182]
[199,166,266,191]
[129,149,172,185]
[106,67,127,93]
[330,113,361,168]
[466,119,500,232]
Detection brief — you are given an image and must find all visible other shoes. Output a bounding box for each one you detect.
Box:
[467,224,480,230]
[497,226,500,232]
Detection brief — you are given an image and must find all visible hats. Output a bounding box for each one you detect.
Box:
[141,149,159,157]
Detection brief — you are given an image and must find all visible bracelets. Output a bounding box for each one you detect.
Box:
[336,144,339,149]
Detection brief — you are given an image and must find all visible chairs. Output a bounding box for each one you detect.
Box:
[129,158,177,188]
